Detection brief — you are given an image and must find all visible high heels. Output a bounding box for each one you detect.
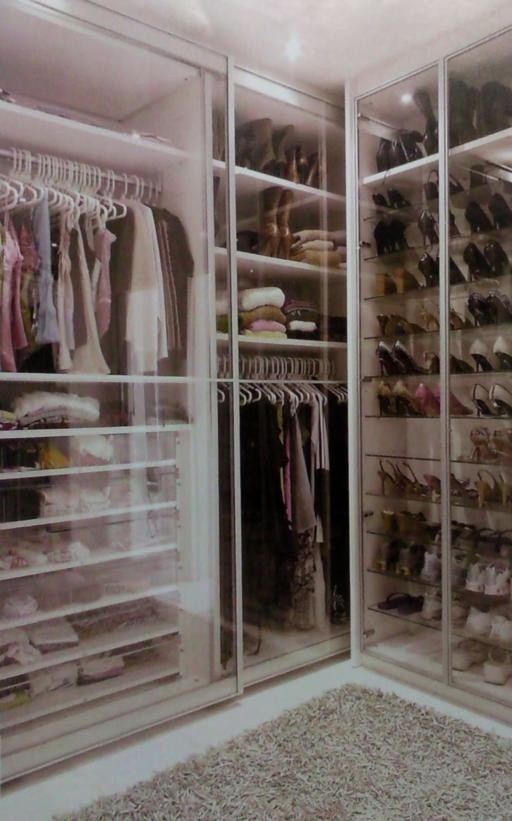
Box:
[373,167,510,417]
[469,427,511,464]
[377,458,511,557]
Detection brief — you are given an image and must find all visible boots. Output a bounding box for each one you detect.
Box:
[349,77,512,169]
[238,117,319,259]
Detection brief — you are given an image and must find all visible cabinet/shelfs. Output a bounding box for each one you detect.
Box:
[209,56,346,701]
[0,2,221,788]
[353,17,511,740]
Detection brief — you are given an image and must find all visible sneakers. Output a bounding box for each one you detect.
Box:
[375,539,512,685]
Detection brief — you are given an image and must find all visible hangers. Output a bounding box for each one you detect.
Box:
[208,352,351,411]
[0,147,180,233]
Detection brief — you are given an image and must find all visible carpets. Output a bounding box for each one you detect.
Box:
[43,684,512,821]
